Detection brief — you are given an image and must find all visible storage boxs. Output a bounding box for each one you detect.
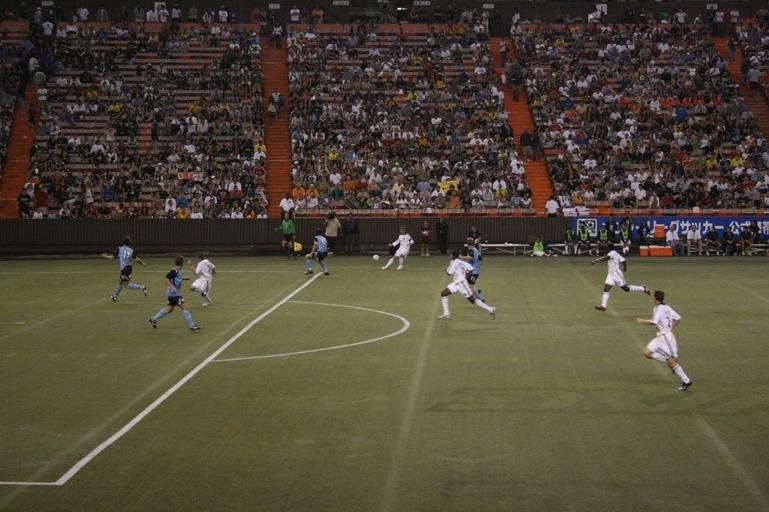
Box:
[638,224,674,257]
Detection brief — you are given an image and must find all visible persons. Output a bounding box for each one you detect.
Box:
[590,242,651,312]
[187,252,216,306]
[636,290,692,391]
[149,256,203,330]
[437,251,496,320]
[458,238,485,303]
[102,236,147,302]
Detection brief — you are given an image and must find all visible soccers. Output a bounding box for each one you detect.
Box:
[372,254,379,261]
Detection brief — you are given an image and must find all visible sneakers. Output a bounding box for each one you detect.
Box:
[142,284,147,296]
[201,300,214,306]
[593,304,607,313]
[489,306,496,321]
[421,253,430,257]
[381,264,403,270]
[149,317,157,328]
[108,293,117,302]
[304,270,330,275]
[435,312,451,319]
[190,323,203,330]
[642,284,650,295]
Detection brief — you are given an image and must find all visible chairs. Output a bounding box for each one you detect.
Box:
[285,21,769,211]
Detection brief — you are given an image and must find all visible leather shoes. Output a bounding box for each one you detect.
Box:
[677,379,693,390]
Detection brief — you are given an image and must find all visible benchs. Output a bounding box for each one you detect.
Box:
[1,18,269,218]
[457,243,629,258]
[683,231,769,255]
